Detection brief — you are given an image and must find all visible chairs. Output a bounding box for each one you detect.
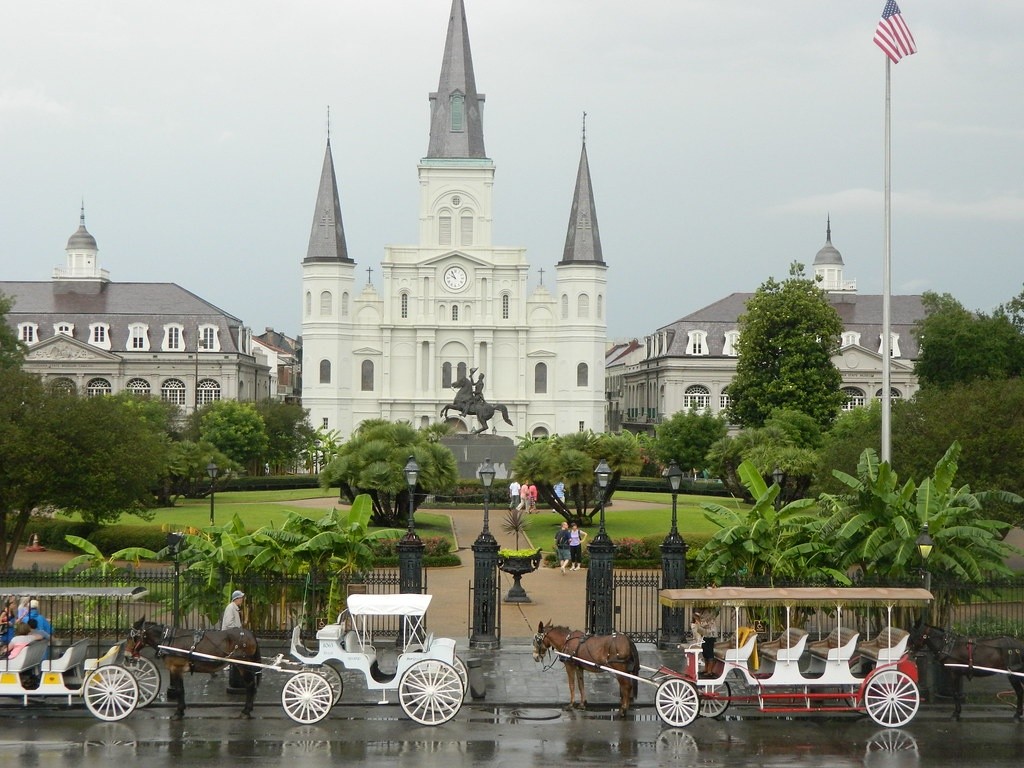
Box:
[345,630,378,668]
[399,632,434,669]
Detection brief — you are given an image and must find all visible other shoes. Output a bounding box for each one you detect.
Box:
[702,672,712,677]
[574,567,580,571]
[570,567,575,570]
[561,567,566,573]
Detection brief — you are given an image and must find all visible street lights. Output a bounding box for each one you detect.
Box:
[206,460,220,526]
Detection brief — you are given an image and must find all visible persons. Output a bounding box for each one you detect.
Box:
[568,523,587,571]
[458,373,484,418]
[552,481,567,513]
[508,480,537,514]
[0,595,55,680]
[554,522,571,575]
[696,605,719,677]
[219,591,247,671]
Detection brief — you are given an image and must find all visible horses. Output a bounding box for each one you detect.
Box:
[440,376,513,435]
[124,614,262,720]
[905,616,1024,724]
[532,620,640,717]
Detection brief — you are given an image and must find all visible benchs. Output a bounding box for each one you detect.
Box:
[803,626,860,680]
[753,627,809,684]
[41,635,88,672]
[713,626,758,682]
[84,638,127,671]
[857,626,910,677]
[0,635,47,672]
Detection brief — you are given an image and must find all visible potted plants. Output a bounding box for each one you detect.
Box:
[497,503,542,603]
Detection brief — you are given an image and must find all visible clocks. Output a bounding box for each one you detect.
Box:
[442,263,470,293]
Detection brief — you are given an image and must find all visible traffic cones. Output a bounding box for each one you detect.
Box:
[26,533,45,552]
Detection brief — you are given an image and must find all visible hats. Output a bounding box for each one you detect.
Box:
[232,591,244,600]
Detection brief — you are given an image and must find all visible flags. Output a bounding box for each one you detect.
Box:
[873,0,918,65]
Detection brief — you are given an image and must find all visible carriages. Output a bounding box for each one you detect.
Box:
[532,588,934,729]
[124,594,470,726]
